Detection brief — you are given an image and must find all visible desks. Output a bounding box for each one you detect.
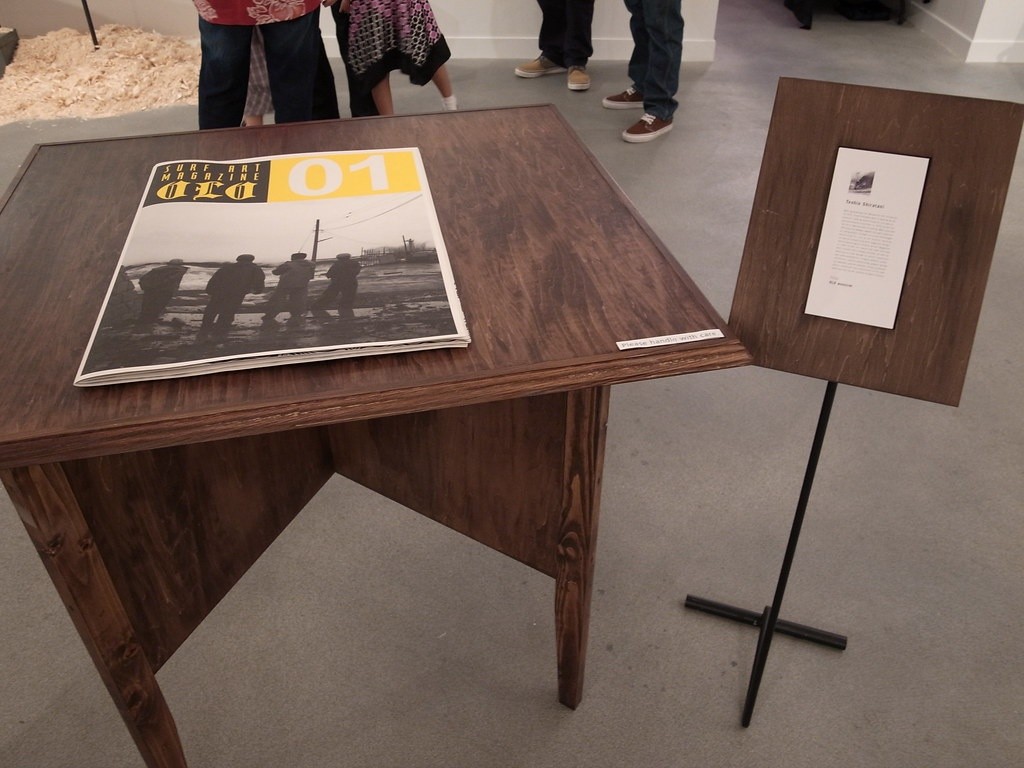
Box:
[0,103,753,768]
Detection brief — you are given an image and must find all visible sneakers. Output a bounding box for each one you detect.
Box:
[622,114,673,142]
[567,65,590,90]
[602,86,644,109]
[514,53,567,77]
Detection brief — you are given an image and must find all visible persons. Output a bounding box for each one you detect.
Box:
[261,252,316,325]
[191,0,342,130]
[515,0,596,93]
[601,0,684,142]
[332,0,456,116]
[310,253,363,320]
[196,254,265,345]
[131,258,188,334]
